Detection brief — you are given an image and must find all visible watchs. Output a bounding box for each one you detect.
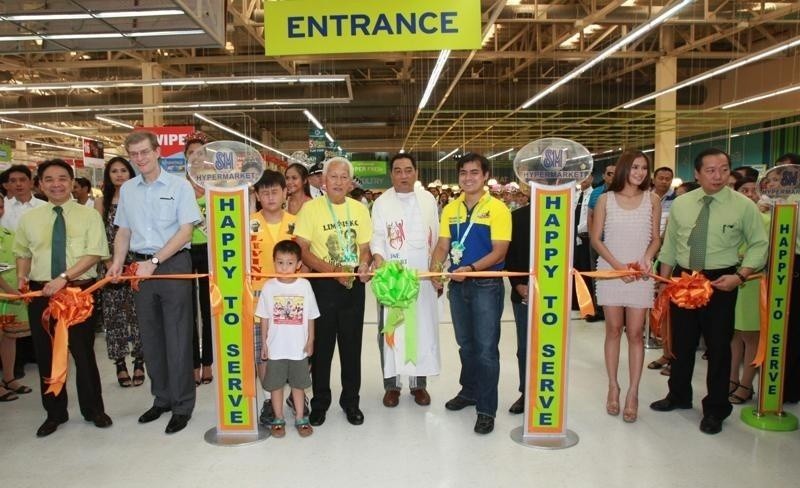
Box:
[59,272,69,283]
[150,257,161,266]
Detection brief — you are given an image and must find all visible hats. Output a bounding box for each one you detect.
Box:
[308,164,324,176]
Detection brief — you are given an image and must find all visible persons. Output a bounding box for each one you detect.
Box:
[0,184,32,401]
[430,153,513,434]
[728,177,771,404]
[71,176,95,209]
[250,169,312,429]
[34,175,48,202]
[345,175,529,209]
[505,156,565,415]
[570,160,596,321]
[0,172,16,207]
[758,164,800,406]
[104,132,204,435]
[308,162,327,199]
[292,156,373,426]
[182,138,216,388]
[12,158,112,438]
[774,153,800,167]
[649,149,771,434]
[241,161,264,212]
[95,157,145,387]
[588,164,617,322]
[589,147,663,424]
[284,163,313,215]
[733,166,759,183]
[0,164,48,380]
[324,234,342,267]
[642,167,677,350]
[726,172,742,191]
[255,239,321,438]
[646,182,700,376]
[343,228,359,263]
[370,153,441,408]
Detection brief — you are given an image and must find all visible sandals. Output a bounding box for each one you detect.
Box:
[0,385,19,402]
[1,378,32,394]
[113,357,131,388]
[132,357,146,386]
[271,418,286,438]
[294,419,313,438]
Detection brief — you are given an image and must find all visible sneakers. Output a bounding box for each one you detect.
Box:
[259,399,275,423]
[383,391,400,407]
[286,390,309,416]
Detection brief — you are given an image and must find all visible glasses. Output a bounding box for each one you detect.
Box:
[129,149,151,157]
[607,172,615,176]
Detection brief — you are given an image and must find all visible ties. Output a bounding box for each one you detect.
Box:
[575,192,584,226]
[687,196,714,273]
[51,206,66,280]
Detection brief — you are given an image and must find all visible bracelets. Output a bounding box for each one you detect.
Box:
[466,264,476,272]
[332,267,337,279]
[360,262,369,271]
[736,272,746,283]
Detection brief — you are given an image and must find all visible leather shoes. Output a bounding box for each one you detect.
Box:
[138,405,172,423]
[165,415,191,433]
[309,409,327,426]
[343,407,364,425]
[36,408,69,437]
[84,413,112,427]
[445,396,477,410]
[410,388,431,406]
[650,398,692,411]
[509,395,524,413]
[474,414,494,434]
[699,414,723,434]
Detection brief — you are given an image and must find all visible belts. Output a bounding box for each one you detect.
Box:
[128,248,188,261]
[36,279,93,287]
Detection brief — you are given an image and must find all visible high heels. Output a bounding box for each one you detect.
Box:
[201,362,214,384]
[661,365,672,374]
[606,388,620,415]
[648,355,671,369]
[623,396,637,423]
[729,380,740,397]
[729,384,755,404]
[193,365,201,387]
